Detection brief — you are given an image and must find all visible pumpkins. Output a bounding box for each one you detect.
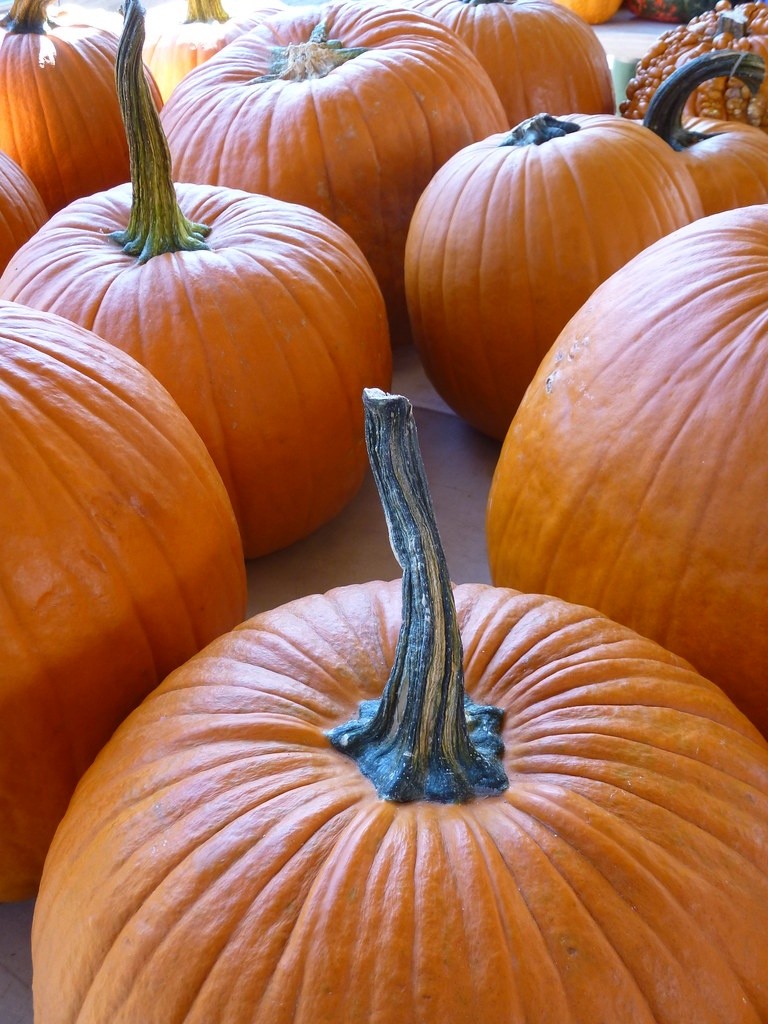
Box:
[0,1,768,1024]
